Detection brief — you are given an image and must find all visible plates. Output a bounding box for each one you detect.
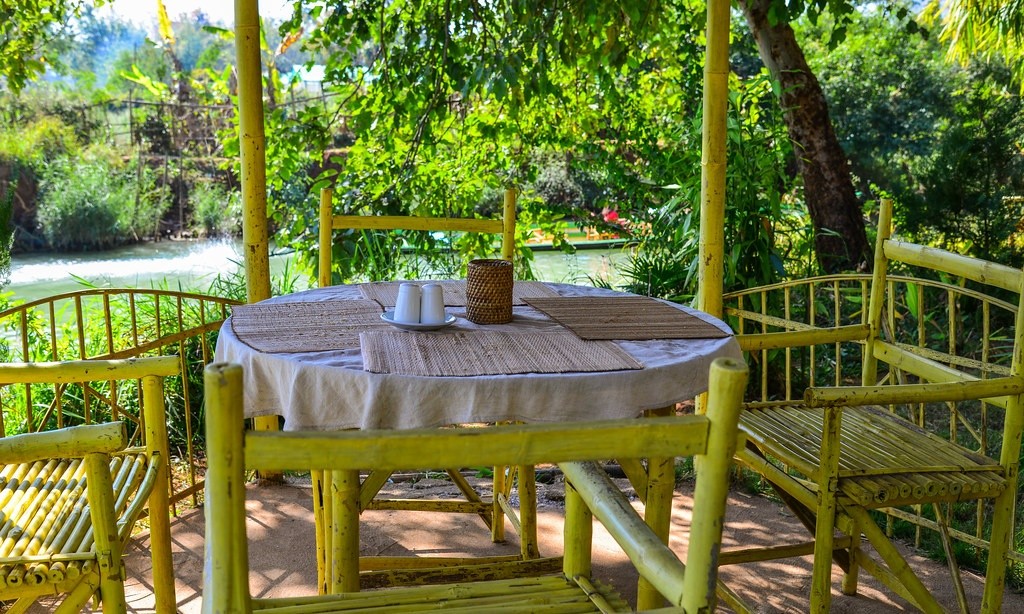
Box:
[380,312,457,331]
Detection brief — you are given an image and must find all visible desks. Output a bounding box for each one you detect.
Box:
[212,280,737,611]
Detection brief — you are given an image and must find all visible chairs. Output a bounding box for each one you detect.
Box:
[306,188,557,596]
[694,197,1024,614]
[0,354,184,614]
[203,357,751,614]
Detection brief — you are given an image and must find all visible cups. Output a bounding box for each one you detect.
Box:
[421,284,444,324]
[393,284,420,324]
